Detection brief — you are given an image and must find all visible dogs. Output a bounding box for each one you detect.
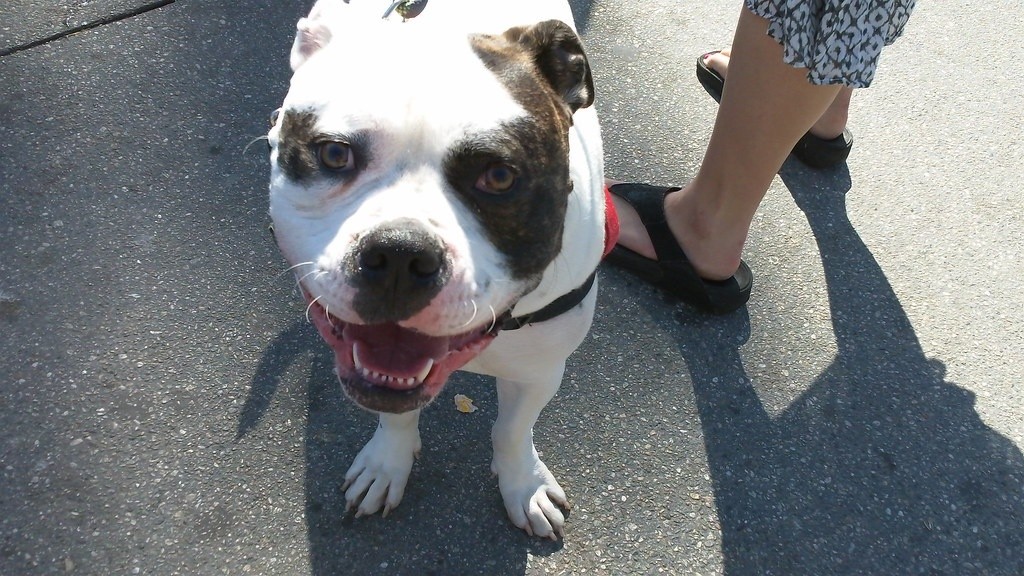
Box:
[240,0,606,542]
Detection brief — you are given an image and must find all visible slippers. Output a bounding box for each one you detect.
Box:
[603,183,759,315]
[697,51,853,169]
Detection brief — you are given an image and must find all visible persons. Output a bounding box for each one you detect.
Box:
[604,1,916,311]
